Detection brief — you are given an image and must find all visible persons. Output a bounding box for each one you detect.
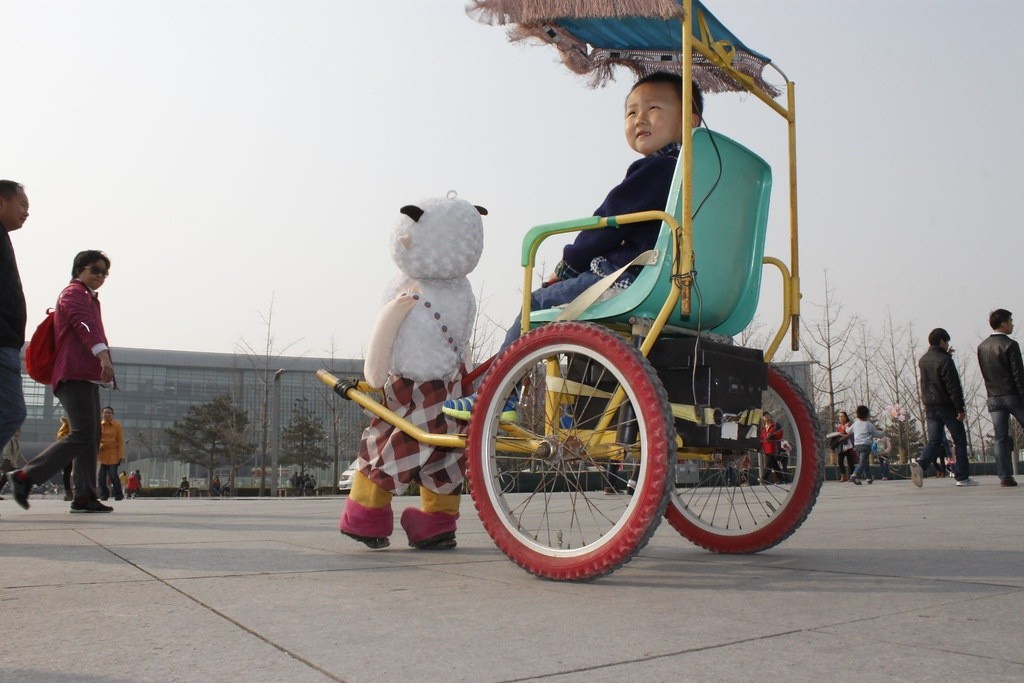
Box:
[908,328,979,487]
[441,70,705,424]
[758,411,792,486]
[209,476,221,497]
[932,439,956,479]
[222,476,233,497]
[603,454,641,496]
[0,406,142,502]
[832,405,892,486]
[174,475,190,498]
[0,179,30,457]
[977,310,1024,486]
[287,471,317,497]
[5,250,116,515]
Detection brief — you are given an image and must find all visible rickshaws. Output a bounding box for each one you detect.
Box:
[316,2,826,584]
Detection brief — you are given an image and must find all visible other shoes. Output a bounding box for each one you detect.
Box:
[936,472,945,478]
[604,486,625,494]
[1001,478,1017,487]
[866,478,872,484]
[840,474,847,482]
[849,474,862,485]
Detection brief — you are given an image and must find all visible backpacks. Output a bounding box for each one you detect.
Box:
[25,283,91,384]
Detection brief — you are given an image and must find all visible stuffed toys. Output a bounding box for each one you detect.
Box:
[338,190,492,552]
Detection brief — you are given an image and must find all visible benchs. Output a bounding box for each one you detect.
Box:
[277,487,324,497]
[209,488,238,496]
[179,487,198,497]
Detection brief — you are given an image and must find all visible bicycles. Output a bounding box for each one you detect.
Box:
[496,462,515,493]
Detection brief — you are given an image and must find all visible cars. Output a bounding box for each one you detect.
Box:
[338,457,358,492]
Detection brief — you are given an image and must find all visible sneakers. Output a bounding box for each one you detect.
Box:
[559,412,574,430]
[910,462,924,487]
[441,391,517,424]
[6,469,33,510]
[956,478,979,486]
[70,501,113,513]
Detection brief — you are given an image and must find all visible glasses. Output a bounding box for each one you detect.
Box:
[84,265,109,278]
